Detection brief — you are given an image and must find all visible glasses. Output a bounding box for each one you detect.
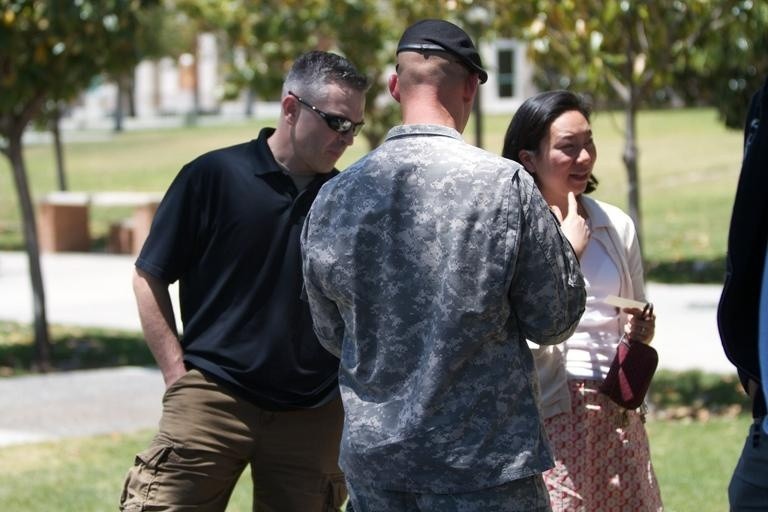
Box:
[288,90,366,136]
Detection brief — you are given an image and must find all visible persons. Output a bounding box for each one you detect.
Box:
[117,49,375,512]
[714,85,768,512]
[296,17,588,512]
[500,90,665,511]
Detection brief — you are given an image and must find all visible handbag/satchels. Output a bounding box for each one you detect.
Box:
[599,336,659,409]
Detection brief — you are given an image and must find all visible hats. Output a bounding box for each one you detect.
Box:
[395,18,489,85]
